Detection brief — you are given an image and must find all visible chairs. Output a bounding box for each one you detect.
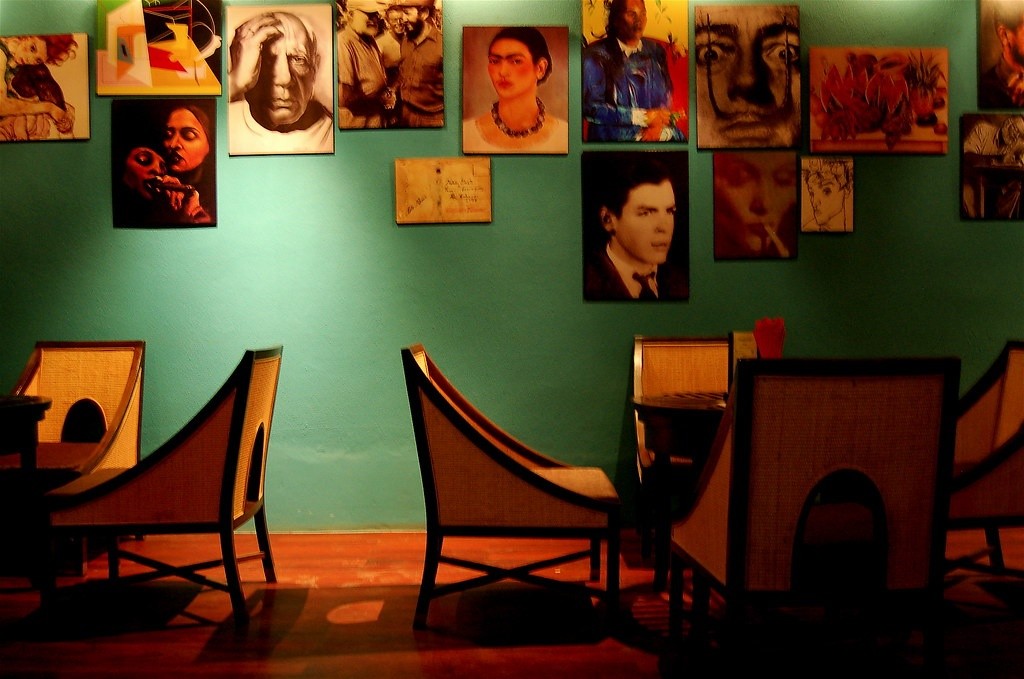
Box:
[629,337,962,679]
[40,346,285,631]
[0,339,145,574]
[400,343,623,633]
[948,341,1024,601]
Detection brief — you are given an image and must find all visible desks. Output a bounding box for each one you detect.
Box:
[0,396,97,630]
[637,392,729,633]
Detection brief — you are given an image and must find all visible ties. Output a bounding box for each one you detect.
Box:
[632,272,658,302]
